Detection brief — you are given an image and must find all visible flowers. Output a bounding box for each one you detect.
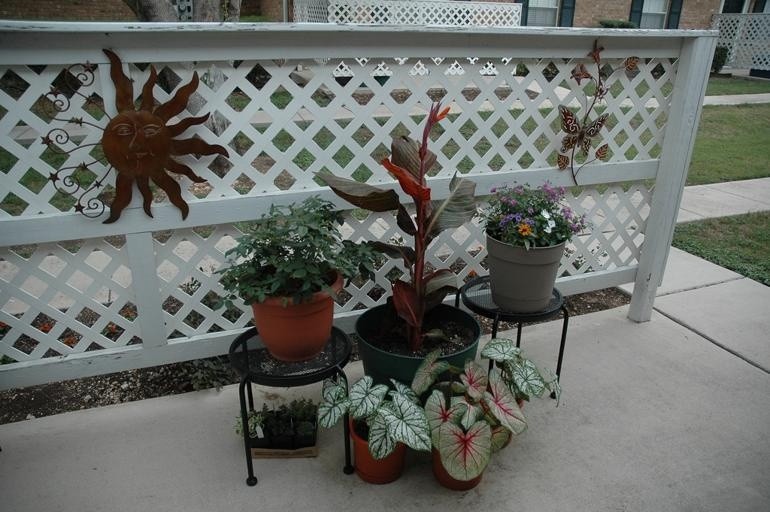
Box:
[476,180,588,252]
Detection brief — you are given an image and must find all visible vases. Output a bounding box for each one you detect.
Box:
[485,230,566,314]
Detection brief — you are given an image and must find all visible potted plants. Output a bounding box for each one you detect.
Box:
[235,397,324,461]
[213,194,378,366]
[410,348,530,491]
[317,368,431,486]
[315,99,483,395]
[482,337,561,451]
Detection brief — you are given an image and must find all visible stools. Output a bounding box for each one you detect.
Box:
[224,322,355,488]
[455,275,569,399]
